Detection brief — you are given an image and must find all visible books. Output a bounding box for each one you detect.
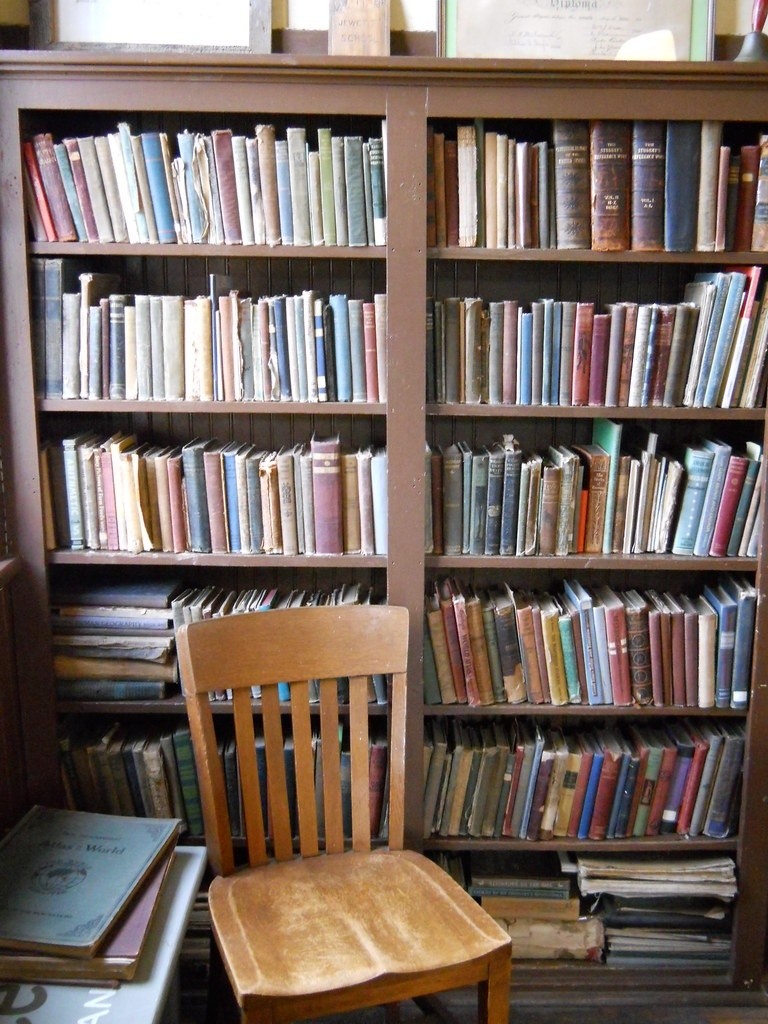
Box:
[19,122,389,930]
[328,0,390,56]
[422,119,768,967]
[0,805,182,988]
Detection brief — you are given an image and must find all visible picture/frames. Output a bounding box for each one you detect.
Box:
[28,0,273,56]
[437,1,716,62]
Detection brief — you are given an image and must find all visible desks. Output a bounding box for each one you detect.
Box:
[1,847,208,1024]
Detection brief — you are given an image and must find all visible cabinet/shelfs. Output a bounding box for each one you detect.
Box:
[2,49,768,993]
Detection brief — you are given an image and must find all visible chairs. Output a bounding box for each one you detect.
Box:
[176,605,511,1024]
